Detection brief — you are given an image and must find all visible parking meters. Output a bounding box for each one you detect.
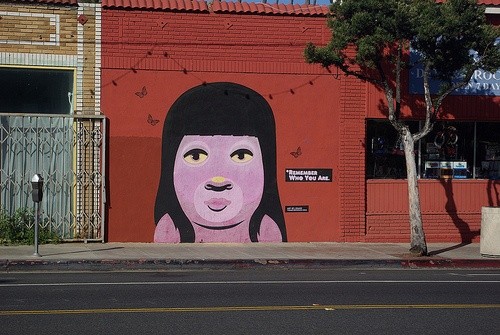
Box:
[30,174,43,258]
